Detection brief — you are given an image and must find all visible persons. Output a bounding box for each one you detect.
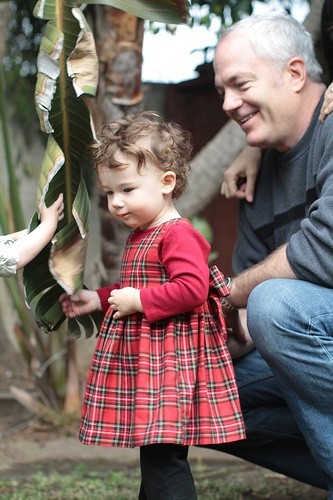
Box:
[213,0,332,201]
[58,110,246,500]
[0,193,64,277]
[193,10,333,491]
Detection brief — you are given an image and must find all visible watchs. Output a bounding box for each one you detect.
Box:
[225,277,231,290]
